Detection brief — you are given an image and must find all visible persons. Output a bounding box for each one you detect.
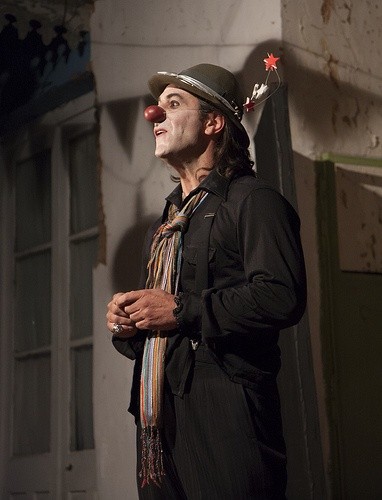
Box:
[105,63,308,500]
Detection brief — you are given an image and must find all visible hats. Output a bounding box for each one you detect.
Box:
[148,63,252,150]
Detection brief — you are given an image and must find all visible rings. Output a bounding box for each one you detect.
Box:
[113,323,121,333]
